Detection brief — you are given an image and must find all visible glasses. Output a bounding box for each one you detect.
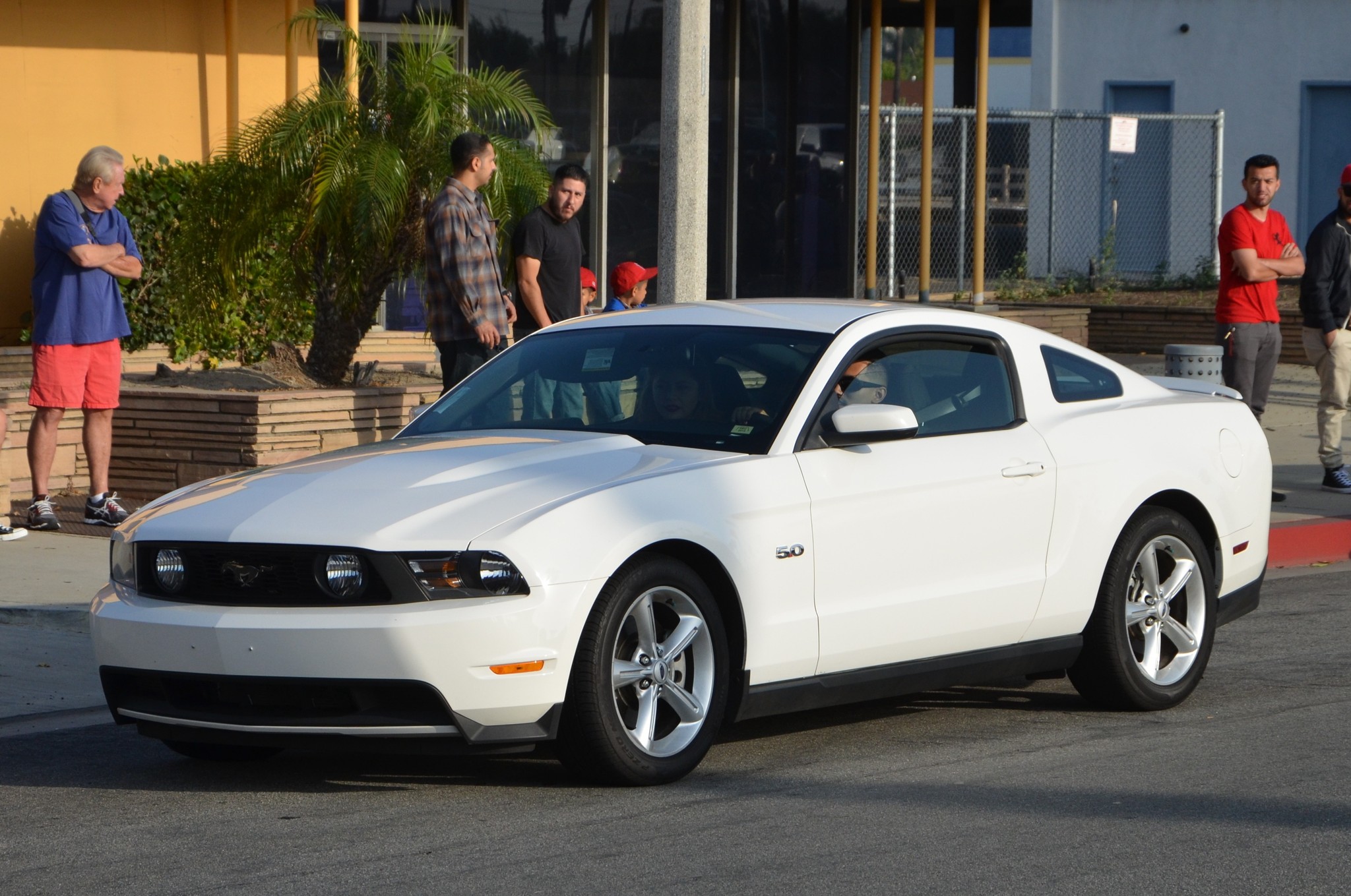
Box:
[1341,184,1351,198]
[838,375,886,394]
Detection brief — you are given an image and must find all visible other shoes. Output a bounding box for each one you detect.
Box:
[1272,490,1286,502]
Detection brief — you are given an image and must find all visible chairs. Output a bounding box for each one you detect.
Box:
[888,364,936,434]
[707,364,748,410]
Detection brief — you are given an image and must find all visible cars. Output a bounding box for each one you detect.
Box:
[85,295,1275,778]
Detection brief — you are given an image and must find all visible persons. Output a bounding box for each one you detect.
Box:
[1215,155,1305,501]
[510,164,591,417]
[733,350,893,437]
[424,132,517,430]
[1297,163,1351,496]
[602,261,658,312]
[1,408,28,541]
[26,145,144,528]
[641,356,718,422]
[580,266,624,426]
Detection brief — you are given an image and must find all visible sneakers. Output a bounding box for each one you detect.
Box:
[84,491,130,527]
[1321,463,1351,493]
[0,525,28,541]
[26,494,61,530]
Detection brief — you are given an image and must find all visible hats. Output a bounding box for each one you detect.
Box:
[1341,164,1351,184]
[579,267,597,291]
[611,262,658,295]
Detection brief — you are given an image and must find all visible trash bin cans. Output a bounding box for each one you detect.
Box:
[1163,343,1225,387]
